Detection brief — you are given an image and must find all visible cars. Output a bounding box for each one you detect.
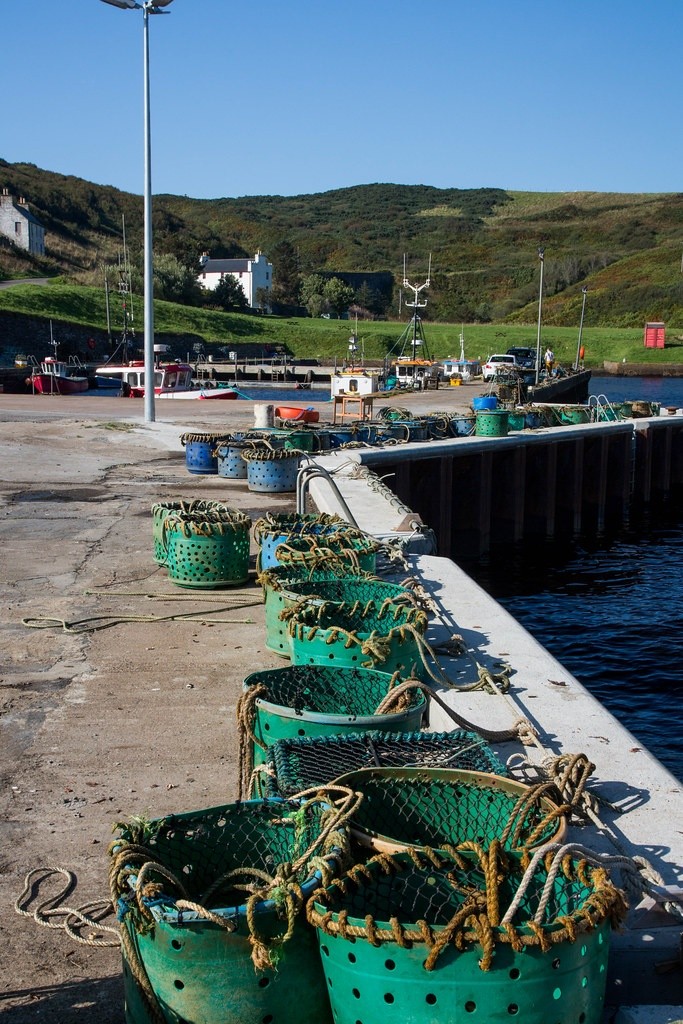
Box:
[484,354,520,381]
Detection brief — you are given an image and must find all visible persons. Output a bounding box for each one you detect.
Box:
[544,347,554,376]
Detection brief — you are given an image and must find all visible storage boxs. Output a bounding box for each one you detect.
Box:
[182,398,658,493]
[115,499,623,1024]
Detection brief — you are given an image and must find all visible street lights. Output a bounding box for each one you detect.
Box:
[97,0,173,430]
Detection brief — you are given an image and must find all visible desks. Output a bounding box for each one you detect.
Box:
[332,396,373,423]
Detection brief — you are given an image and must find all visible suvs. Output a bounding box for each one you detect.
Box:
[506,346,536,368]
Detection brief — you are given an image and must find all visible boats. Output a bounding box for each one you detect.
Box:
[276,406,321,422]
[96,360,241,400]
[28,355,89,394]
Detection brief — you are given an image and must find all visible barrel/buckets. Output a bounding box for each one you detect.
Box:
[473,397,497,410]
